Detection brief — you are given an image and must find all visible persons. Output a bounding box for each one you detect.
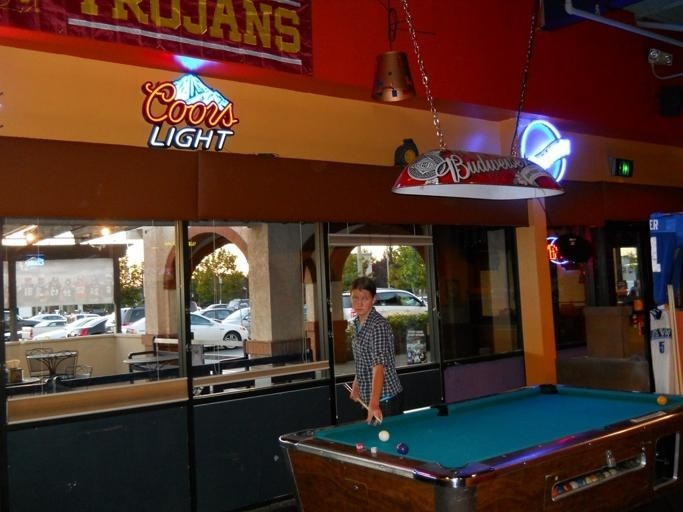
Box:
[348,275,405,427]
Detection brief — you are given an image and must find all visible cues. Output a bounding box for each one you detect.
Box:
[668,284,683,396]
[343,383,382,422]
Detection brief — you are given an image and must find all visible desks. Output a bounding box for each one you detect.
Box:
[277,385,683,509]
[122,355,180,381]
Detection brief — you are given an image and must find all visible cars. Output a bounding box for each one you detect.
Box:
[1,283,250,351]
[342,289,429,323]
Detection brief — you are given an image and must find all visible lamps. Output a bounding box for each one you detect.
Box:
[392,1,567,199]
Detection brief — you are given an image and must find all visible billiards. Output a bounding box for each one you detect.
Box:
[378,430,390,442]
[552,467,617,498]
[656,396,667,405]
[396,442,408,455]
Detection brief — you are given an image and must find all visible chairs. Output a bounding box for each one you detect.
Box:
[128,350,155,385]
[191,344,205,364]
[26,347,92,378]
